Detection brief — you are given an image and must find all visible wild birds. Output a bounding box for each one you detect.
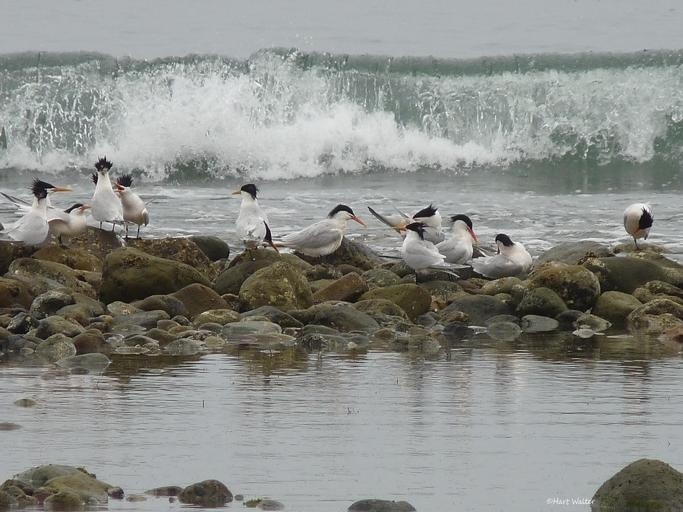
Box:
[467,232,533,281]
[0,174,92,257]
[231,183,279,254]
[112,173,150,240]
[89,154,124,232]
[622,201,655,251]
[280,204,366,259]
[366,203,479,279]
